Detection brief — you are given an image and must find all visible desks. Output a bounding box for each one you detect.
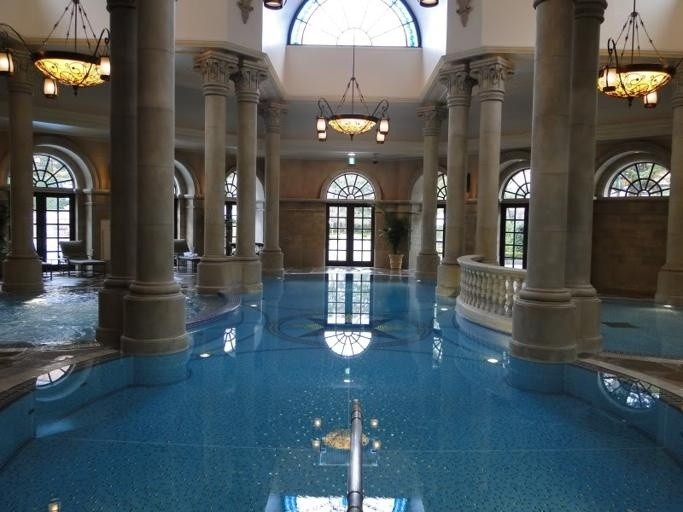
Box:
[69,259,105,275]
[178,255,202,273]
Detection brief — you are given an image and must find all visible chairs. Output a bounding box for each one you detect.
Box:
[173,239,190,271]
[59,240,88,275]
[40,257,53,280]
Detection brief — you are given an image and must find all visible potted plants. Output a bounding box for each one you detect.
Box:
[369,199,410,269]
[383,269,411,320]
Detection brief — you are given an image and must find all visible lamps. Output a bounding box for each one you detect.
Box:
[597,0,682,111]
[309,363,385,454]
[263,0,284,9]
[0,0,112,100]
[419,0,439,7]
[317,46,390,144]
